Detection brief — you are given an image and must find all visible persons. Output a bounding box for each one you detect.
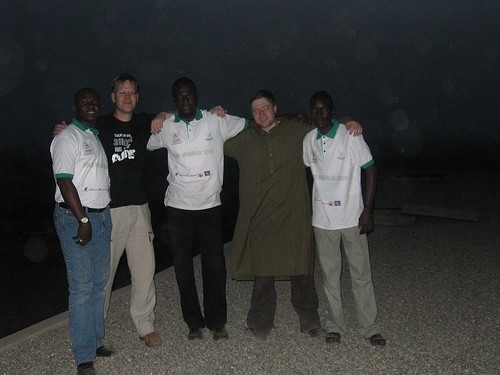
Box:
[53,76,228,347]
[147,78,364,341]
[303,91,388,348]
[50,87,113,375]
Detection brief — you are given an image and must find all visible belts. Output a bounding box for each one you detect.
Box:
[59,203,106,213]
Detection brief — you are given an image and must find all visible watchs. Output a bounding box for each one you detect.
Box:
[78,217,90,224]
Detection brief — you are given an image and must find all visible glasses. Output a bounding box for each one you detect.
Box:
[115,90,138,97]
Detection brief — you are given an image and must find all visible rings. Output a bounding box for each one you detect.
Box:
[80,239,83,243]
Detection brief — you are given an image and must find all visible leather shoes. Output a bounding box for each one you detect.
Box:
[77,360,94,375]
[364,334,386,346]
[140,332,160,346]
[94,346,111,357]
[189,327,202,341]
[212,326,228,344]
[326,332,341,343]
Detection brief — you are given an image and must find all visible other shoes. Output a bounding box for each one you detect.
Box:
[308,327,320,339]
[244,327,256,337]
